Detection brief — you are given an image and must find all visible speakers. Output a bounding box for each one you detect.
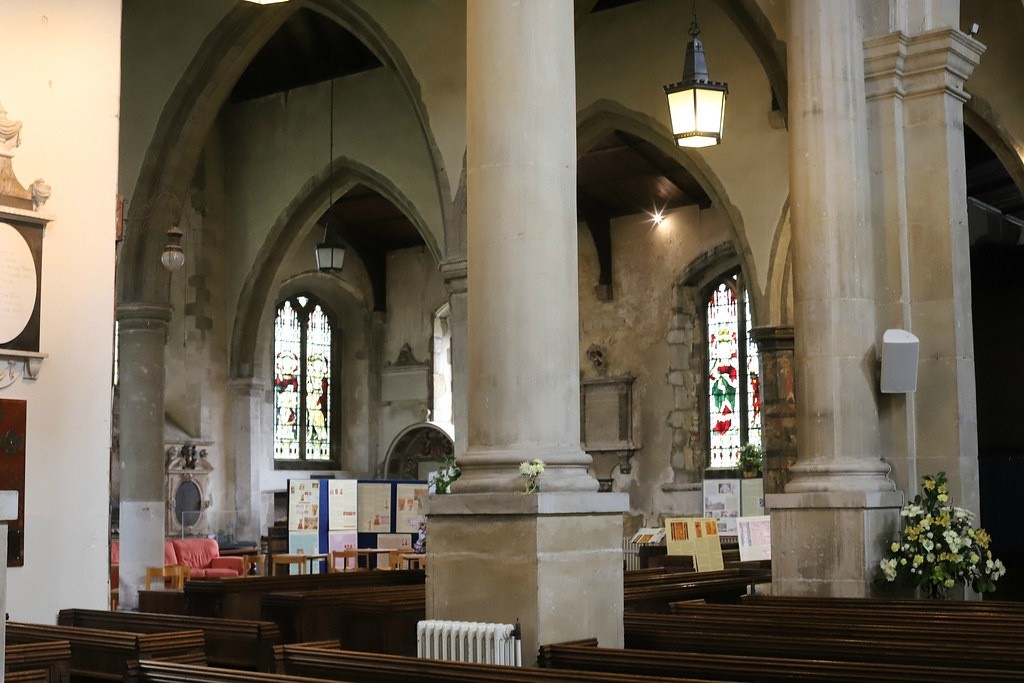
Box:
[879,328,919,394]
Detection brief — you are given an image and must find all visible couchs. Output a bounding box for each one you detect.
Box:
[109,539,251,589]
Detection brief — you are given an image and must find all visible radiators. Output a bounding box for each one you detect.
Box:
[417,618,521,668]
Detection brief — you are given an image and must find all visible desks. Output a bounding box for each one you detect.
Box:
[398,553,426,570]
[272,552,330,577]
[333,548,396,573]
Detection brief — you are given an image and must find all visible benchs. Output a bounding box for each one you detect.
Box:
[4,561,1023,683]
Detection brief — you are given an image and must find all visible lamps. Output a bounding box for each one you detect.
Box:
[664,0,729,147]
[313,70,347,274]
[154,192,185,271]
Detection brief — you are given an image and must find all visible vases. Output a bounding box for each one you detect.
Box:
[743,469,758,478]
[525,477,543,494]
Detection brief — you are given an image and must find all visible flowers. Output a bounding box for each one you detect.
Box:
[435,454,462,494]
[732,443,763,475]
[875,470,1006,599]
[518,460,546,491]
